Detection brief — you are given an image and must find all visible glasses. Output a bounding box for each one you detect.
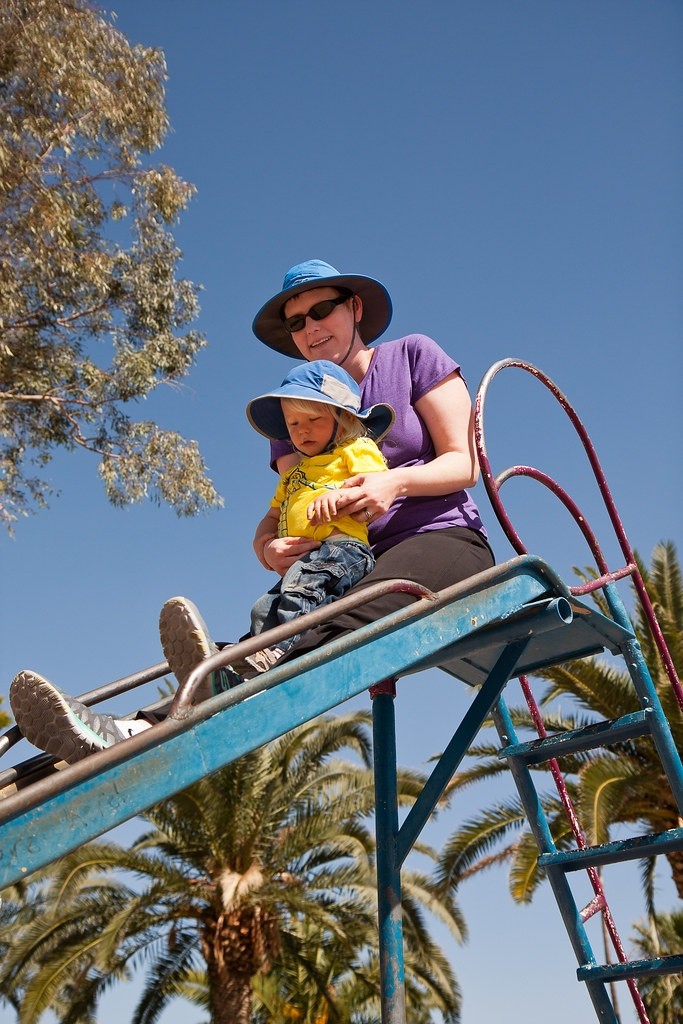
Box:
[284,292,357,334]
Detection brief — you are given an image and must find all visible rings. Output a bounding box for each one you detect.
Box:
[365,511,372,519]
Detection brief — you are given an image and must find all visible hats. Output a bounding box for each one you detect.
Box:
[246,360,396,444]
[252,259,393,359]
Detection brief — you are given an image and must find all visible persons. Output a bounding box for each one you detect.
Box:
[222,360,395,680]
[10,256,498,762]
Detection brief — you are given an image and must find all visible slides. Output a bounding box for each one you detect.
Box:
[0,552,550,893]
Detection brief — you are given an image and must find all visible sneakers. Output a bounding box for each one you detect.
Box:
[223,644,277,679]
[159,596,245,705]
[9,670,126,765]
